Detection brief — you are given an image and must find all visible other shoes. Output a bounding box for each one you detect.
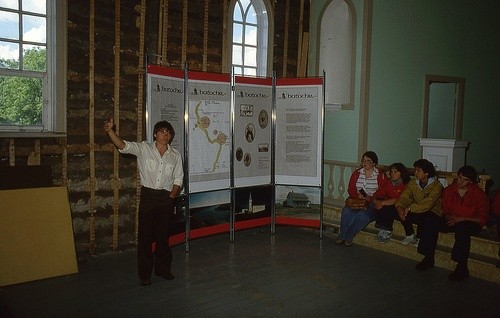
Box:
[344,241,352,246]
[455,269,466,279]
[416,260,434,268]
[142,277,151,285]
[335,239,341,243]
[155,271,174,280]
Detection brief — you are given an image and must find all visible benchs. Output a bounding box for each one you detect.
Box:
[322,200,500,286]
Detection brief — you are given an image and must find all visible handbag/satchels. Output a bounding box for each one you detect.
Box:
[347,196,370,210]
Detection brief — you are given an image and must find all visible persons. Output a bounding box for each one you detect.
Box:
[236,149,250,166]
[335,152,381,246]
[373,163,411,243]
[105,118,184,286]
[491,187,500,235]
[416,166,489,280]
[395,159,444,247]
[260,111,268,128]
[246,124,254,141]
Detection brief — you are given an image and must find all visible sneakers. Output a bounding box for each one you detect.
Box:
[417,239,420,246]
[401,233,416,245]
[374,231,382,240]
[379,231,392,242]
